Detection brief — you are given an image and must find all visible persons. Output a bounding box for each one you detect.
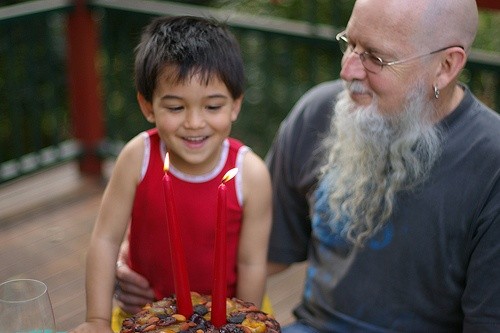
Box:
[113,0,500,332]
[74,15,273,333]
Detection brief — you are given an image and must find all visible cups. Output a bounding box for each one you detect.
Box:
[0,279,56,333]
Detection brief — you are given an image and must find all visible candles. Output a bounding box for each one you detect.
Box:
[210,168,239,329]
[162,151,194,320]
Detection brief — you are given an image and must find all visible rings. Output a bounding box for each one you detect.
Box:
[114,280,123,291]
[113,288,123,300]
[115,259,127,270]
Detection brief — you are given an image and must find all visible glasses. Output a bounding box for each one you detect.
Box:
[335,29,464,74]
